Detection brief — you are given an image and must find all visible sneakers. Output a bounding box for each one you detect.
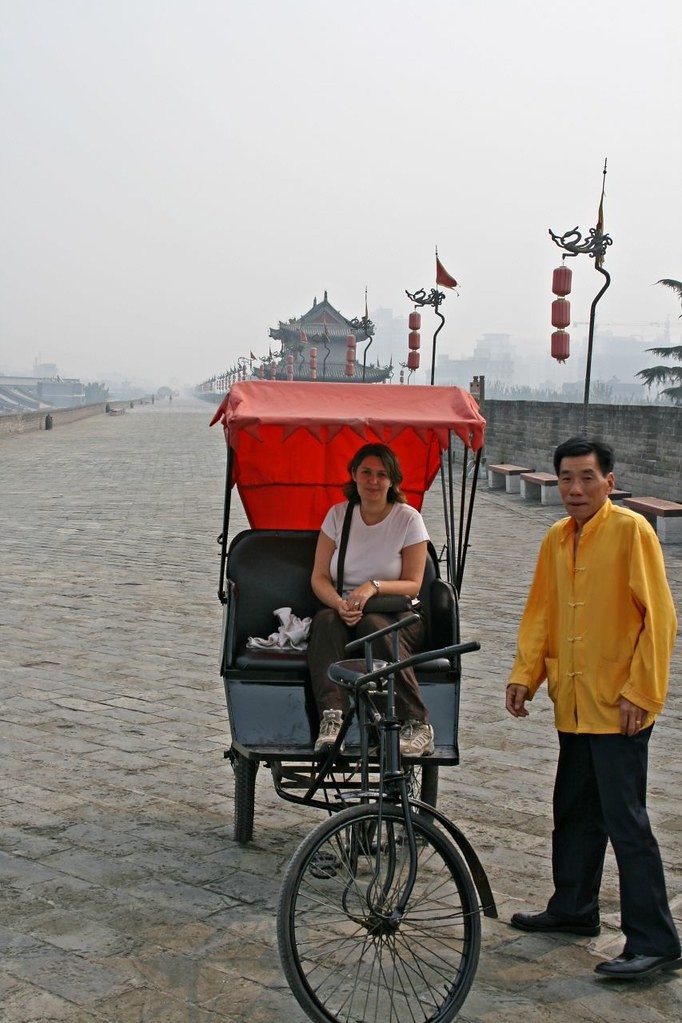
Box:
[315,709,345,752]
[397,719,436,756]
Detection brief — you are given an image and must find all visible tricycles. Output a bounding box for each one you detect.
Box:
[217,380,499,1023]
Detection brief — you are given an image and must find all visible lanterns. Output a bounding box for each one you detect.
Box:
[260,364,264,381]
[552,266,572,363]
[310,347,317,379]
[287,355,293,381]
[345,335,356,375]
[408,312,421,369]
[271,361,276,380]
[217,365,247,389]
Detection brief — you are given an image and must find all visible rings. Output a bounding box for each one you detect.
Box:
[353,601,360,607]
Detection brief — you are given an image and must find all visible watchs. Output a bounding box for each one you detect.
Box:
[369,578,381,596]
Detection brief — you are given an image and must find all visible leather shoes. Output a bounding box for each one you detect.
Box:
[510,907,602,937]
[593,949,682,977]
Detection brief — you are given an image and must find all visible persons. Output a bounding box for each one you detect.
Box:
[306,443,435,758]
[506,435,682,977]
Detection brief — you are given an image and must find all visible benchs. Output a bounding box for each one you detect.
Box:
[622,496,682,545]
[519,471,562,506]
[609,489,632,506]
[488,464,533,494]
[221,528,462,685]
